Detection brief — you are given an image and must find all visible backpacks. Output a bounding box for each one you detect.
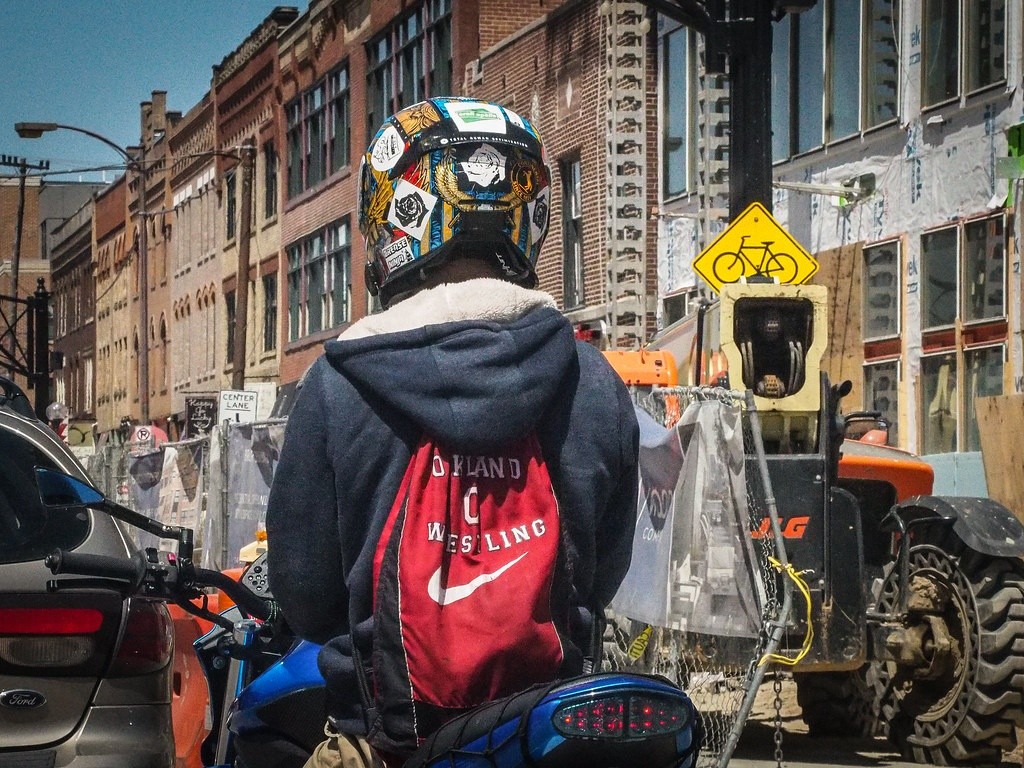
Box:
[365,429,590,761]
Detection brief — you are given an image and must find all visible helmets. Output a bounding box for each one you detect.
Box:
[355,97,552,309]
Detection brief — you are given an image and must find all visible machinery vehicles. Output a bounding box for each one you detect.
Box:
[587,275,1024,768]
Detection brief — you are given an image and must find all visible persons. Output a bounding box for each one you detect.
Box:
[226,95,640,768]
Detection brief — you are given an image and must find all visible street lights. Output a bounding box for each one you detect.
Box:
[15,122,149,428]
[47,402,68,438]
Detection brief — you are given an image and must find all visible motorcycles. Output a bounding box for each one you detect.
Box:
[34,464,707,768]
